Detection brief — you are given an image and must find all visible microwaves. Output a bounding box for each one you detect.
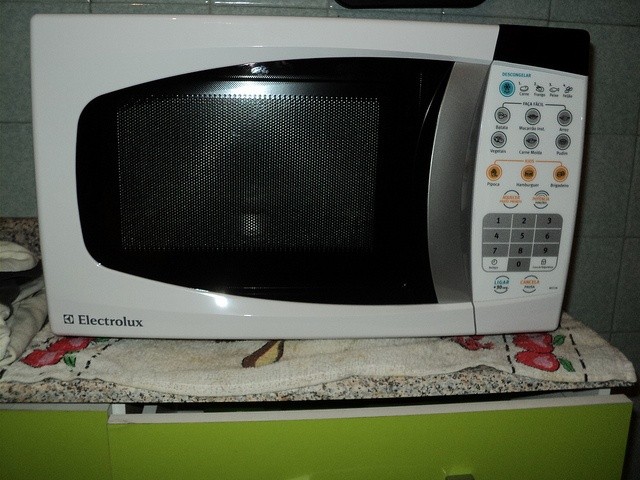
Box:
[30,12,591,342]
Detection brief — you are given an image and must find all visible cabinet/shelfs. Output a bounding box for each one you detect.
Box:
[1,398,112,479]
[106,385,635,479]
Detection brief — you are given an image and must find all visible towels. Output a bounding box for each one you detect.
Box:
[17,327,636,393]
